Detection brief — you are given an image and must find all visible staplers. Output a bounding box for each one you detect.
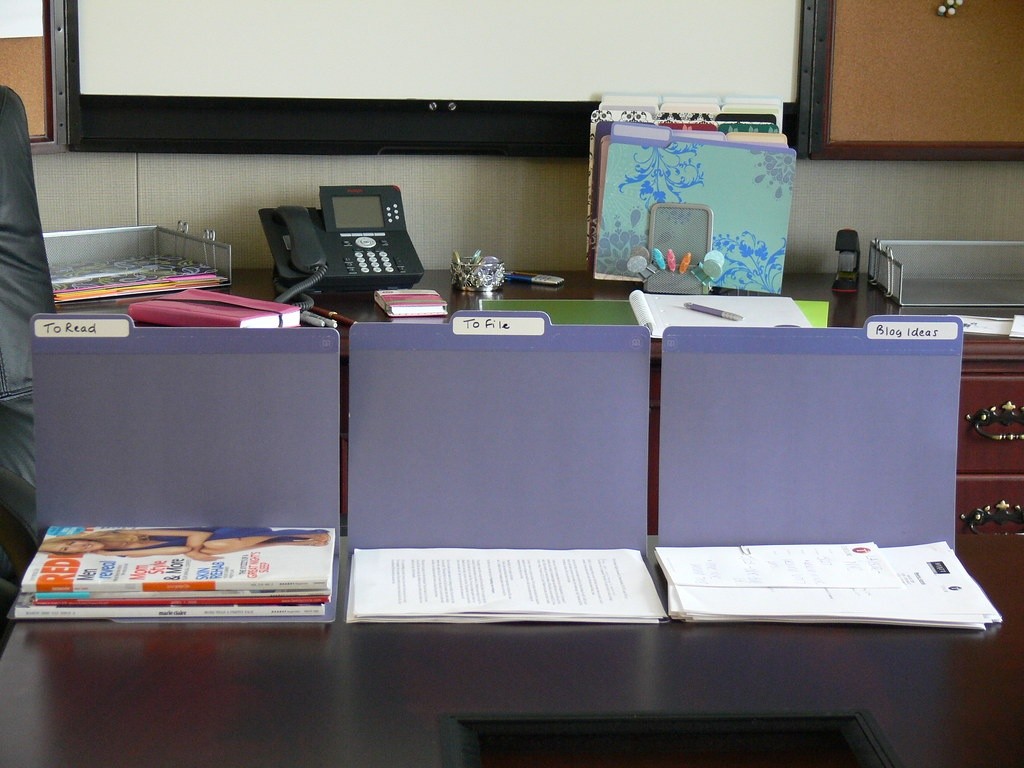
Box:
[830,230,860,292]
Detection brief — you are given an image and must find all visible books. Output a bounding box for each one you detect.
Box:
[374,289,448,317]
[14,525,336,620]
[129,288,301,330]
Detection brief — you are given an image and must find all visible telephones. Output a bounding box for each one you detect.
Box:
[257,185,425,293]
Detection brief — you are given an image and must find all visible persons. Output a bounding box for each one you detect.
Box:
[38,527,331,562]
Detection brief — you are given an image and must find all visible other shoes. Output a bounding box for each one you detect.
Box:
[308,540,327,546]
[313,533,331,542]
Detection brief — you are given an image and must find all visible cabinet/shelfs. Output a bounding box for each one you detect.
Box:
[1,265,1024,768]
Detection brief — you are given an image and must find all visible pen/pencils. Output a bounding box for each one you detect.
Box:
[300,311,338,329]
[311,306,357,327]
[684,302,743,321]
[505,271,564,285]
